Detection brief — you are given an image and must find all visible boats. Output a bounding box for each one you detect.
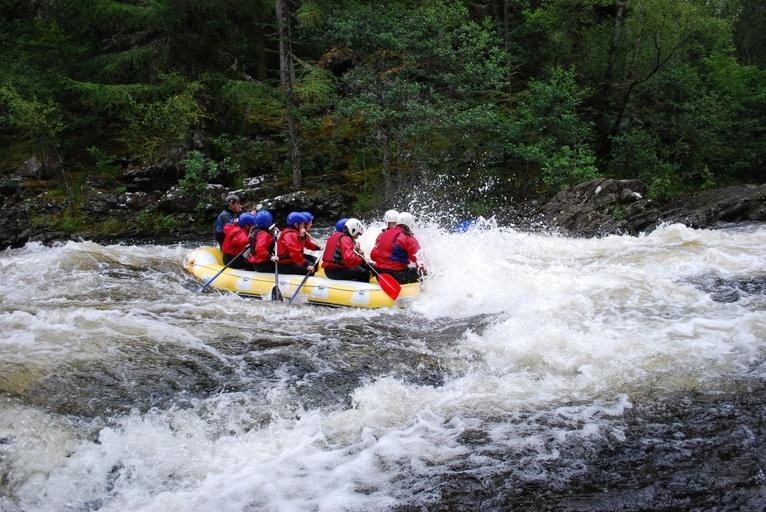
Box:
[181,244,424,311]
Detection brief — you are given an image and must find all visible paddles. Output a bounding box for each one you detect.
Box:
[352,248,401,300]
[191,223,276,294]
[272,230,283,301]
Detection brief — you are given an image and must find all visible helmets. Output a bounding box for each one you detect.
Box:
[253,210,274,229]
[382,209,399,223]
[335,218,349,233]
[344,217,365,237]
[238,212,255,227]
[300,211,315,225]
[285,211,308,227]
[396,211,416,232]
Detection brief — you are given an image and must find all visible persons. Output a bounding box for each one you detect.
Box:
[374,212,427,284]
[277,212,316,276]
[247,211,283,273]
[335,218,364,255]
[321,218,370,282]
[222,213,255,271]
[371,210,399,262]
[214,195,256,249]
[299,212,324,272]
